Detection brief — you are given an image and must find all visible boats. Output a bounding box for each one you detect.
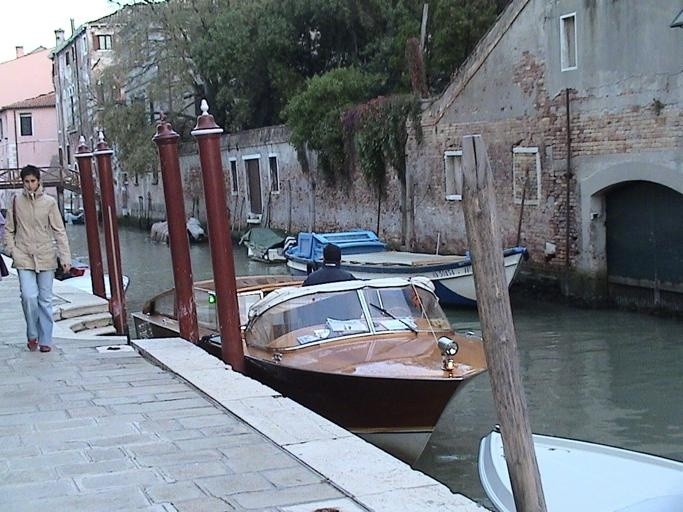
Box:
[478,425,682,512]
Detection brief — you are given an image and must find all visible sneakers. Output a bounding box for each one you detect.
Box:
[40,345,50,352]
[28,337,37,351]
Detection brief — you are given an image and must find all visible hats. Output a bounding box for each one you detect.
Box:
[323,245,341,261]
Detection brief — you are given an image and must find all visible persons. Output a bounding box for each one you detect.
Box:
[0,211,9,281]
[2,164,72,353]
[296,241,364,331]
